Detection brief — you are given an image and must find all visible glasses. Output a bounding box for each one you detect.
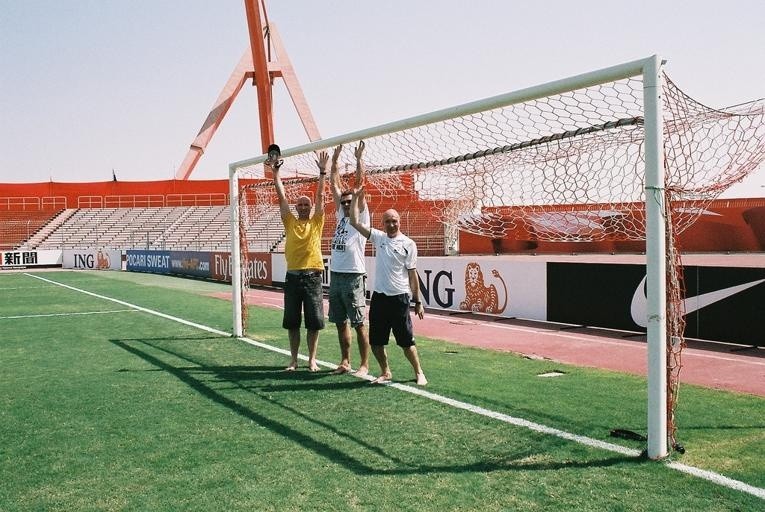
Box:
[339,199,352,205]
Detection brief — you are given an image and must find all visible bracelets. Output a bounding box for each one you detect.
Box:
[415,300,422,305]
[319,172,326,176]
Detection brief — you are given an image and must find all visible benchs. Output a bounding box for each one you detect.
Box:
[1,207,447,256]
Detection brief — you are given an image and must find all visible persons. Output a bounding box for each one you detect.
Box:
[326,139,370,378]
[349,179,430,387]
[268,149,330,373]
